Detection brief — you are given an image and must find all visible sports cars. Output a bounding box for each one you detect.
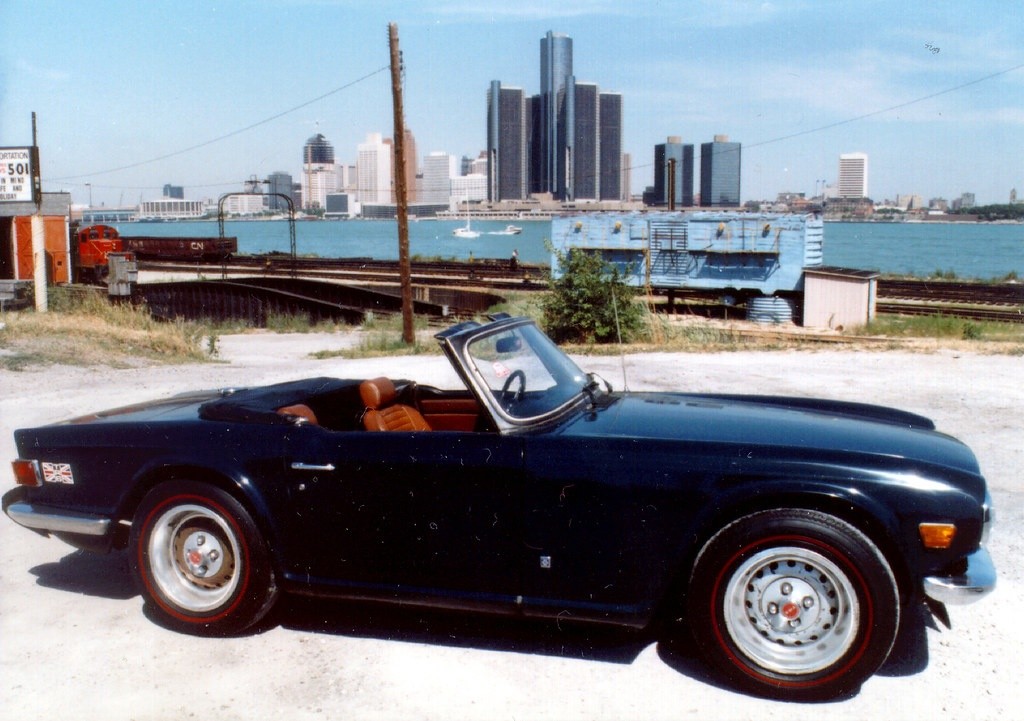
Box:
[1,314,999,705]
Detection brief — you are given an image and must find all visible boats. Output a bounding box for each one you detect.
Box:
[502,225,523,235]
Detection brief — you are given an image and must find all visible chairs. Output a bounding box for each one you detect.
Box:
[360,376,432,432]
[277,402,320,427]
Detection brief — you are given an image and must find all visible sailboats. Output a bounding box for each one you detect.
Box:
[453,186,479,238]
